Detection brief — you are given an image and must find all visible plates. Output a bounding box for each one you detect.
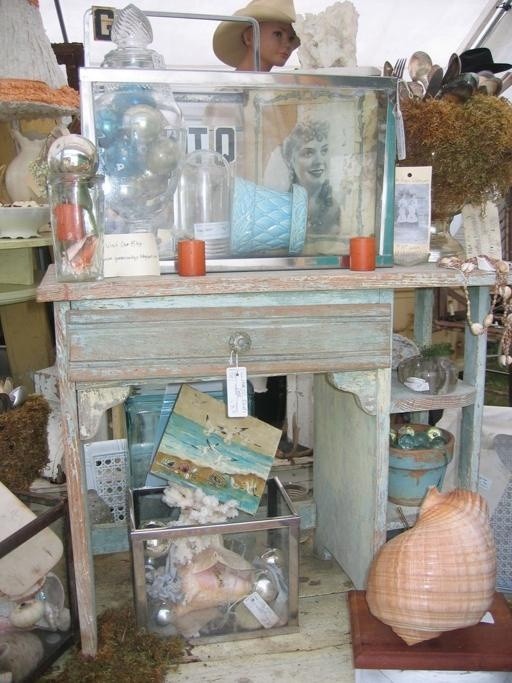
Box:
[34,256,509,658]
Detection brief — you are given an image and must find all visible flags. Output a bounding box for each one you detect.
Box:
[3,129,52,205]
[388,423,455,508]
[231,175,310,256]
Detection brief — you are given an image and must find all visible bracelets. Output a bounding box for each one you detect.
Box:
[1,202,52,239]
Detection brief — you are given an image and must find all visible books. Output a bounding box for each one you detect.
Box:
[128,474,298,644]
[76,63,400,272]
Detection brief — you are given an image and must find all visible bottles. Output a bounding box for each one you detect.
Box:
[213,0,301,68]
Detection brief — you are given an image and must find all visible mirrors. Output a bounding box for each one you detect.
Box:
[192,393,208,408]
[190,413,262,467]
[229,476,258,496]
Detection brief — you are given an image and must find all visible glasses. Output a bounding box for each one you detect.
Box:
[1,0,81,152]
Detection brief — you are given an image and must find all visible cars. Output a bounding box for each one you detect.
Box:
[350,236,377,272]
[45,171,107,283]
[177,239,206,278]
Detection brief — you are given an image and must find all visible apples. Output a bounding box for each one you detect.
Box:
[349,235,378,271]
[178,237,207,277]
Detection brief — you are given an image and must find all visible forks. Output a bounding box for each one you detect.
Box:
[393,56,407,79]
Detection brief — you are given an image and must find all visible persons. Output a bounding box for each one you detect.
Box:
[194,0,306,178]
[278,117,351,235]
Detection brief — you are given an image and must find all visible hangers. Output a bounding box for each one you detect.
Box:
[384,61,393,77]
